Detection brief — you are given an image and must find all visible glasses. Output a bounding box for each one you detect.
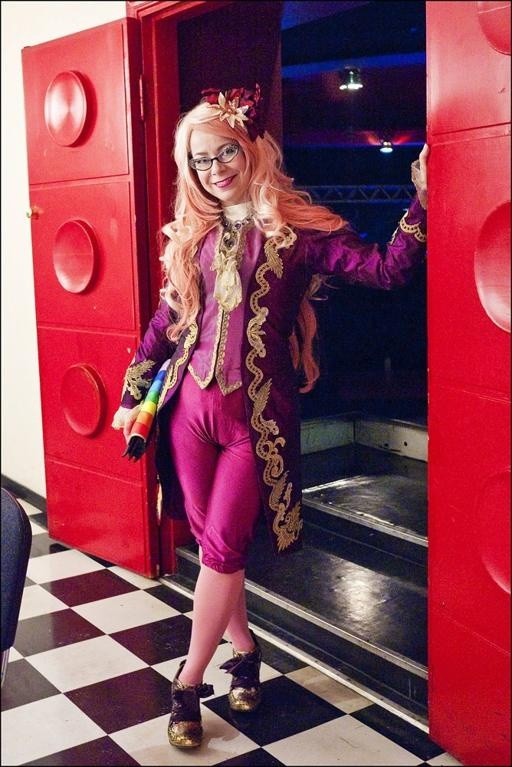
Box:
[188,144,239,171]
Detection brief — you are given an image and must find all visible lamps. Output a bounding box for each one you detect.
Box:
[378,139,396,154]
[337,64,366,92]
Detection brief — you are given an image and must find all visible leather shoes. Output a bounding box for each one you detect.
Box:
[225,626,263,713]
[167,658,203,749]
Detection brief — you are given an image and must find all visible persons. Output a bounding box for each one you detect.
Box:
[112,87,428,750]
